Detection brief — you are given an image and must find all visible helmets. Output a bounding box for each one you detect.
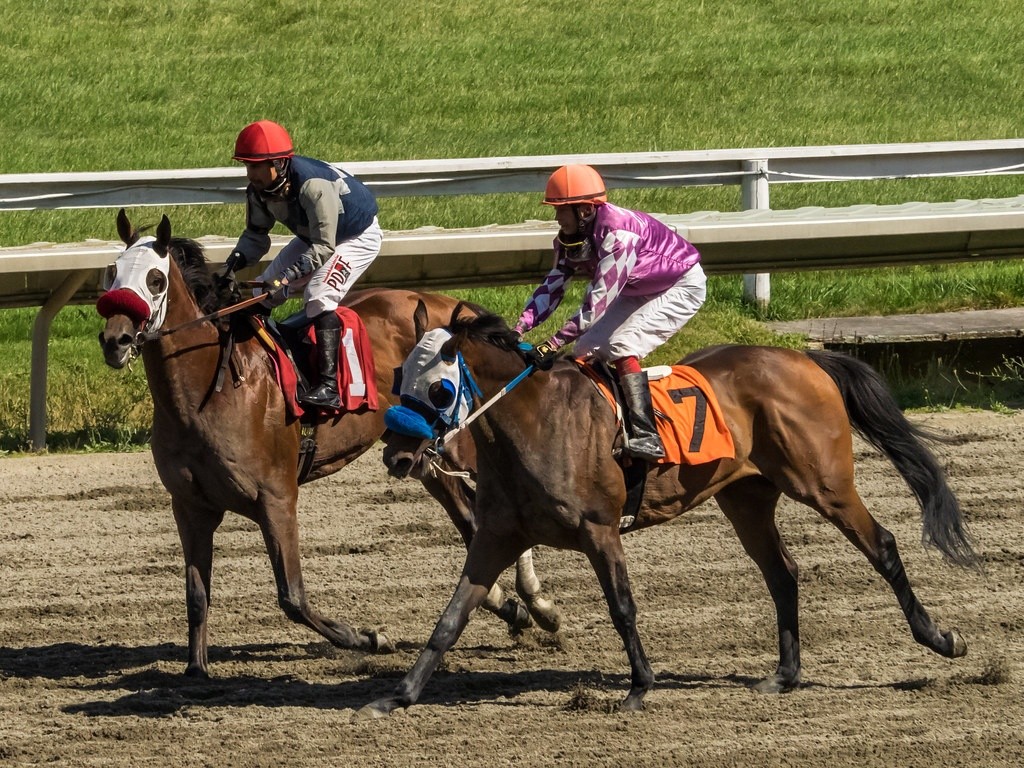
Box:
[542,164,608,205]
[231,120,294,162]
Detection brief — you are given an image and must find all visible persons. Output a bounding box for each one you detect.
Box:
[513,164,706,462]
[213,119,382,409]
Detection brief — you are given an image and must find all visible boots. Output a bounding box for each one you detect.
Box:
[611,371,666,460]
[299,326,342,409]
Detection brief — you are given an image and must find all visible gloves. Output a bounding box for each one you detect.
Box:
[260,271,292,302]
[526,341,558,370]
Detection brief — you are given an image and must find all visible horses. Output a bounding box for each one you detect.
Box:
[382,298,987,712]
[95,207,562,685]
